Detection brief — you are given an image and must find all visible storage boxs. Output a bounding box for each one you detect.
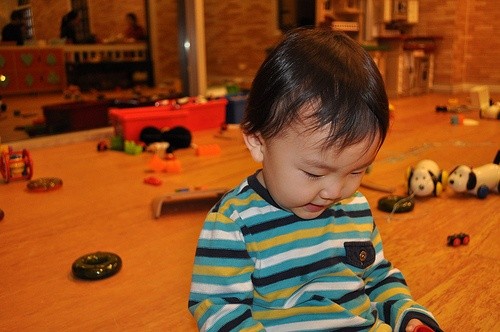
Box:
[41,101,108,133]
[107,92,248,145]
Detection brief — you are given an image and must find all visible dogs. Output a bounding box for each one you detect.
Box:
[406,159,447,198]
[447,148,500,199]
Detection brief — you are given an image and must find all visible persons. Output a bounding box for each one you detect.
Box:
[58,8,95,44]
[187,26,445,332]
[119,11,145,41]
[1,9,24,42]
